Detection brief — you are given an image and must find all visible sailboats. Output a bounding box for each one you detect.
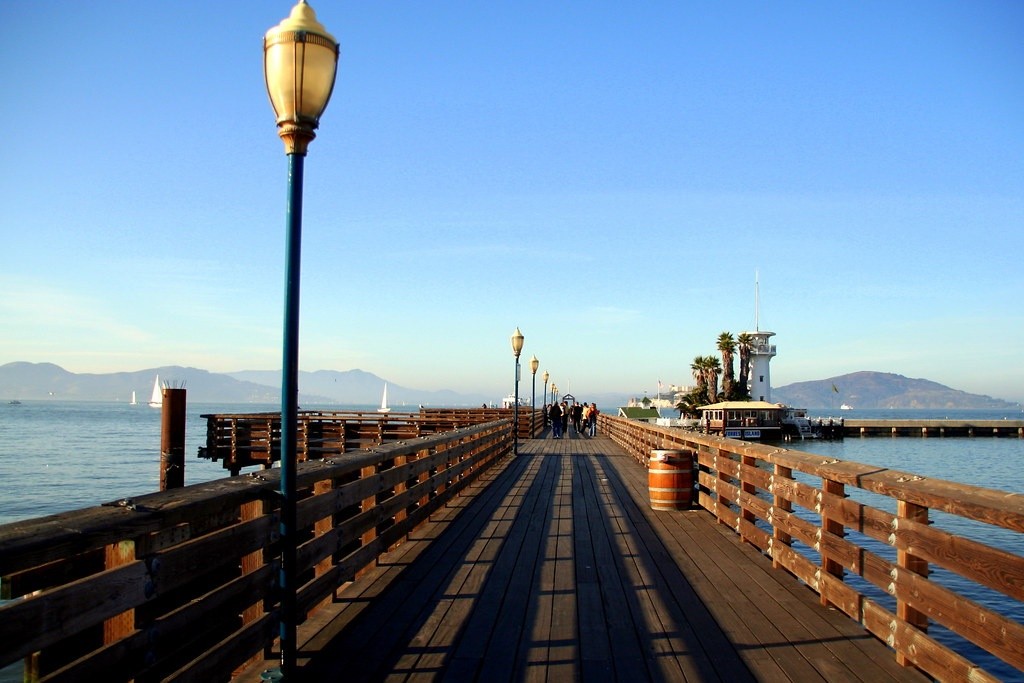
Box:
[377,383,391,412]
[147,374,163,408]
[129,390,137,404]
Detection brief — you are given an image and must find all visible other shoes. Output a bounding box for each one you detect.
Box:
[553,436,560,439]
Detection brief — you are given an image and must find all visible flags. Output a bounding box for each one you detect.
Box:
[832,384,838,393]
[659,381,664,388]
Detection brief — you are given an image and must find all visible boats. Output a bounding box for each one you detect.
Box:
[841,402,854,409]
[8,400,21,404]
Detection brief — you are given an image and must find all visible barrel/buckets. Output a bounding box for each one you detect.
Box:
[648,448,695,513]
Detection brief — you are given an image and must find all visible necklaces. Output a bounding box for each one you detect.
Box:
[547,404,552,426]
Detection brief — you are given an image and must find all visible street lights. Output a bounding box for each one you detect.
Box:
[542,370,549,430]
[528,352,539,439]
[510,325,524,456]
[261,0,341,683]
[550,381,559,406]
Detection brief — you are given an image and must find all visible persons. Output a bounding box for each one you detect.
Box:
[482,404,486,409]
[570,402,600,438]
[508,404,512,409]
[549,400,562,439]
[560,401,570,435]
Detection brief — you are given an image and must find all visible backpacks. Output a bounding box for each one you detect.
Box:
[589,409,596,418]
